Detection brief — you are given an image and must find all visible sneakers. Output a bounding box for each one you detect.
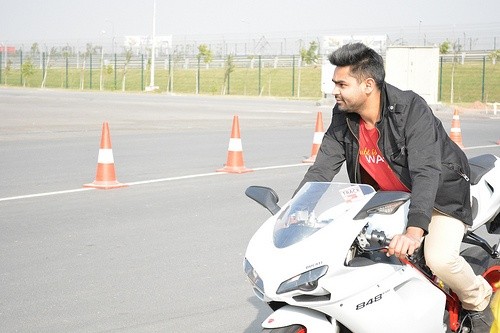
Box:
[464,292,499,333]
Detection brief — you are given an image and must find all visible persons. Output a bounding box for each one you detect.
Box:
[284,42,496,333]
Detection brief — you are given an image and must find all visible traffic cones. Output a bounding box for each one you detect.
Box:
[84,123,127,189]
[447,109,464,150]
[302,112,325,163]
[216,115,255,174]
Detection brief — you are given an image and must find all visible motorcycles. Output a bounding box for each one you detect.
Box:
[242,151,500,333]
